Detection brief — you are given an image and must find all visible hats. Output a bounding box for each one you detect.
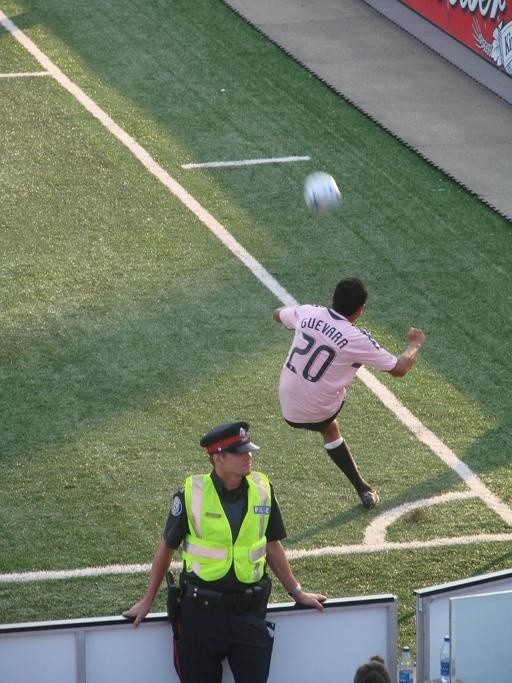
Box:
[199,421,261,456]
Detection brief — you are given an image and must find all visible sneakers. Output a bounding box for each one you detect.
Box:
[360,488,381,508]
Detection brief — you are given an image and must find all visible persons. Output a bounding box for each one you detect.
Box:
[275,278,425,508]
[353,655,391,683]
[123,421,325,683]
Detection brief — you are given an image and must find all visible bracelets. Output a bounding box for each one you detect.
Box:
[287,584,302,598]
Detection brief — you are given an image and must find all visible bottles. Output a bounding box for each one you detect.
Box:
[399,646,413,683]
[439,635,456,682]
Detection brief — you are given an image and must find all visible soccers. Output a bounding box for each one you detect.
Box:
[304,171,339,213]
